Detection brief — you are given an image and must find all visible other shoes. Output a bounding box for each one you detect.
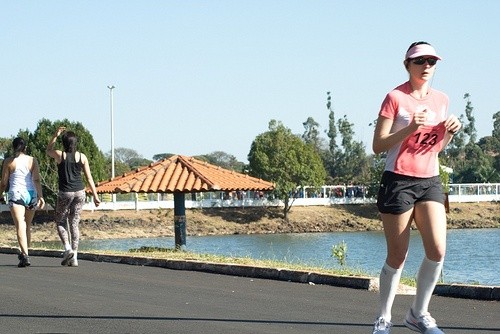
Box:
[18,257,31,267]
[18,251,23,259]
[65,259,78,266]
[61,250,74,265]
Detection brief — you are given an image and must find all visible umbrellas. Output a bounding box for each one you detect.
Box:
[83,154,281,250]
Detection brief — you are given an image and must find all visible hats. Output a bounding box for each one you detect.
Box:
[406,44,442,61]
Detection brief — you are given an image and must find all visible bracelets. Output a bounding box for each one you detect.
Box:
[37,196,43,198]
[448,130,456,135]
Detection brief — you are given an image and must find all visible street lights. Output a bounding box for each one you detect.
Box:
[107,85,117,200]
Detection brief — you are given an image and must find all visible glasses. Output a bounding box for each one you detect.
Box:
[407,56,437,65]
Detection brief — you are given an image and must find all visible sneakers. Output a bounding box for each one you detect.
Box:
[404,309,444,334]
[372,313,392,334]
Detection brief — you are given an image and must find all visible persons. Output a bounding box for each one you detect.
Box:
[0,137,45,268]
[46,127,101,268]
[370,42,461,334]
[224,185,366,200]
